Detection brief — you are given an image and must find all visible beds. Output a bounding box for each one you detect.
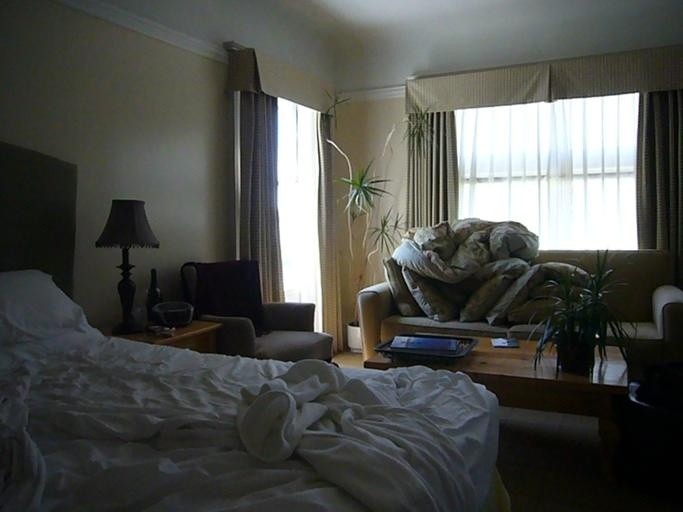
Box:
[0,144,502,512]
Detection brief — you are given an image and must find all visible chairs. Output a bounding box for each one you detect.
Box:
[183,263,336,362]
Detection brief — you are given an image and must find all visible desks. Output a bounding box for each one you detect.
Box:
[364,338,630,418]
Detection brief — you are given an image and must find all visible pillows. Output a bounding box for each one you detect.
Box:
[381,256,591,325]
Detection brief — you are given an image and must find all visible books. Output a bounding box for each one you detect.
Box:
[390,335,458,354]
[491,338,519,348]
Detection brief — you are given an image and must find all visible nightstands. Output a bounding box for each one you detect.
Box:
[103,318,224,354]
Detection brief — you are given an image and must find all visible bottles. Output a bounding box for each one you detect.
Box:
[146,268,164,322]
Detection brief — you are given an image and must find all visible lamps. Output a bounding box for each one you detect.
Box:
[95,200,159,337]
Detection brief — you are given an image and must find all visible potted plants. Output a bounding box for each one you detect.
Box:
[525,250,640,373]
[322,83,435,354]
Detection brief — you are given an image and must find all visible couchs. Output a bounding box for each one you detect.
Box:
[357,250,683,379]
